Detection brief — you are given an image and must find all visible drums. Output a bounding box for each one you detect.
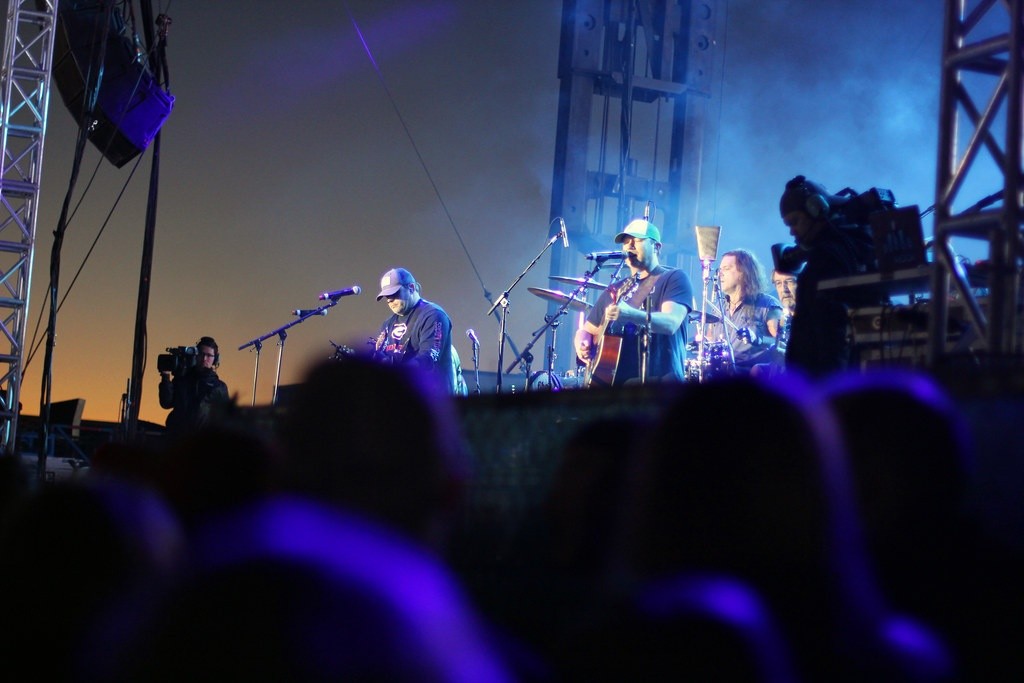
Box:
[523,369,591,391]
[685,341,730,383]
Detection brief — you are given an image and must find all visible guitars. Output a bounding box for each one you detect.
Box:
[369,327,388,356]
[584,272,641,386]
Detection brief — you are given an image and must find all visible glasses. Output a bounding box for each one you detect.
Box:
[196,351,216,359]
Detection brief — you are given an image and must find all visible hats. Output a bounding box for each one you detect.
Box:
[376,268,415,301]
[614,219,662,247]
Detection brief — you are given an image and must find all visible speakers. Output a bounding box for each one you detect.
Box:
[36,0,176,169]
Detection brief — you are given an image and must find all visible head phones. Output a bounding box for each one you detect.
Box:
[196,336,220,365]
[786,175,831,220]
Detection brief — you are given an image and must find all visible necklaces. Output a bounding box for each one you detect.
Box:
[730,300,740,311]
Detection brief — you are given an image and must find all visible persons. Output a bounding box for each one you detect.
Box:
[573,219,692,381]
[686,249,797,377]
[159,336,226,429]
[346,266,454,397]
[0,350,1024,683]
[779,176,889,382]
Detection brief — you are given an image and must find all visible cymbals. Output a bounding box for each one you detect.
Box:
[689,308,720,324]
[526,286,594,310]
[549,275,607,291]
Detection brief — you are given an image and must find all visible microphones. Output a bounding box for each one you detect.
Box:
[584,250,629,260]
[345,349,356,358]
[466,329,480,347]
[712,269,719,303]
[643,203,650,221]
[560,218,569,248]
[292,308,327,316]
[319,286,361,300]
